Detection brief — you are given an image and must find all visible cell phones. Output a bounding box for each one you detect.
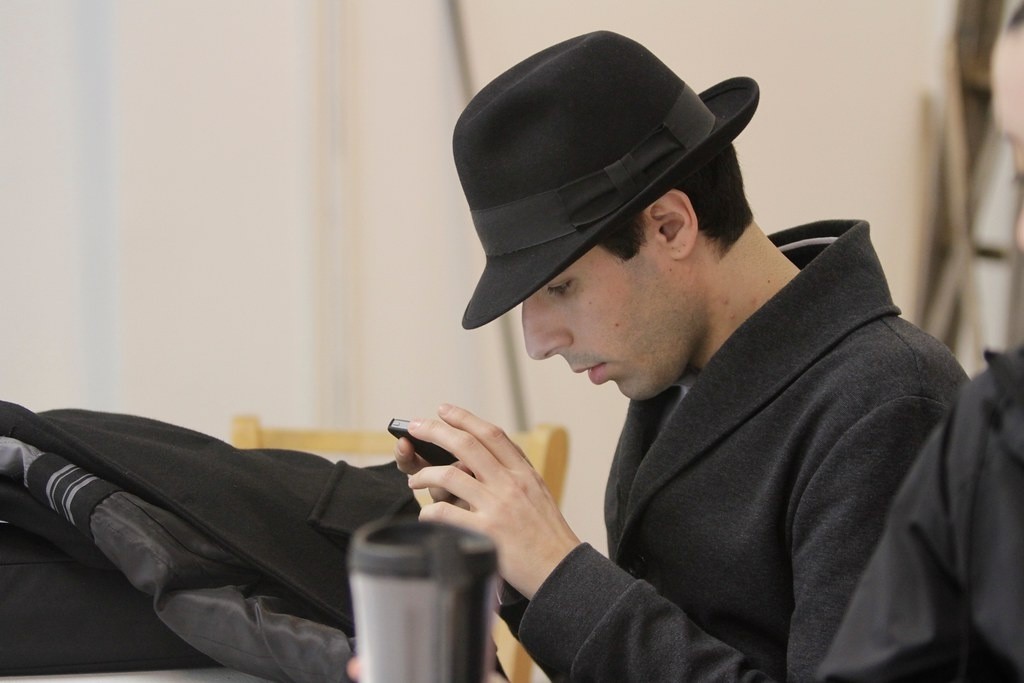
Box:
[387,417,459,467]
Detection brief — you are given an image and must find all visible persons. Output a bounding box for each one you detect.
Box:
[813,1,1024,683]
[395,30,975,683]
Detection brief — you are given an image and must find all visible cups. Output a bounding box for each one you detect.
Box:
[352,518,497,683]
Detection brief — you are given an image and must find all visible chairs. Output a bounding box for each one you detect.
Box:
[233,416,568,683]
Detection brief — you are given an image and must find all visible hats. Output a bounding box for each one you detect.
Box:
[451,30,760,331]
